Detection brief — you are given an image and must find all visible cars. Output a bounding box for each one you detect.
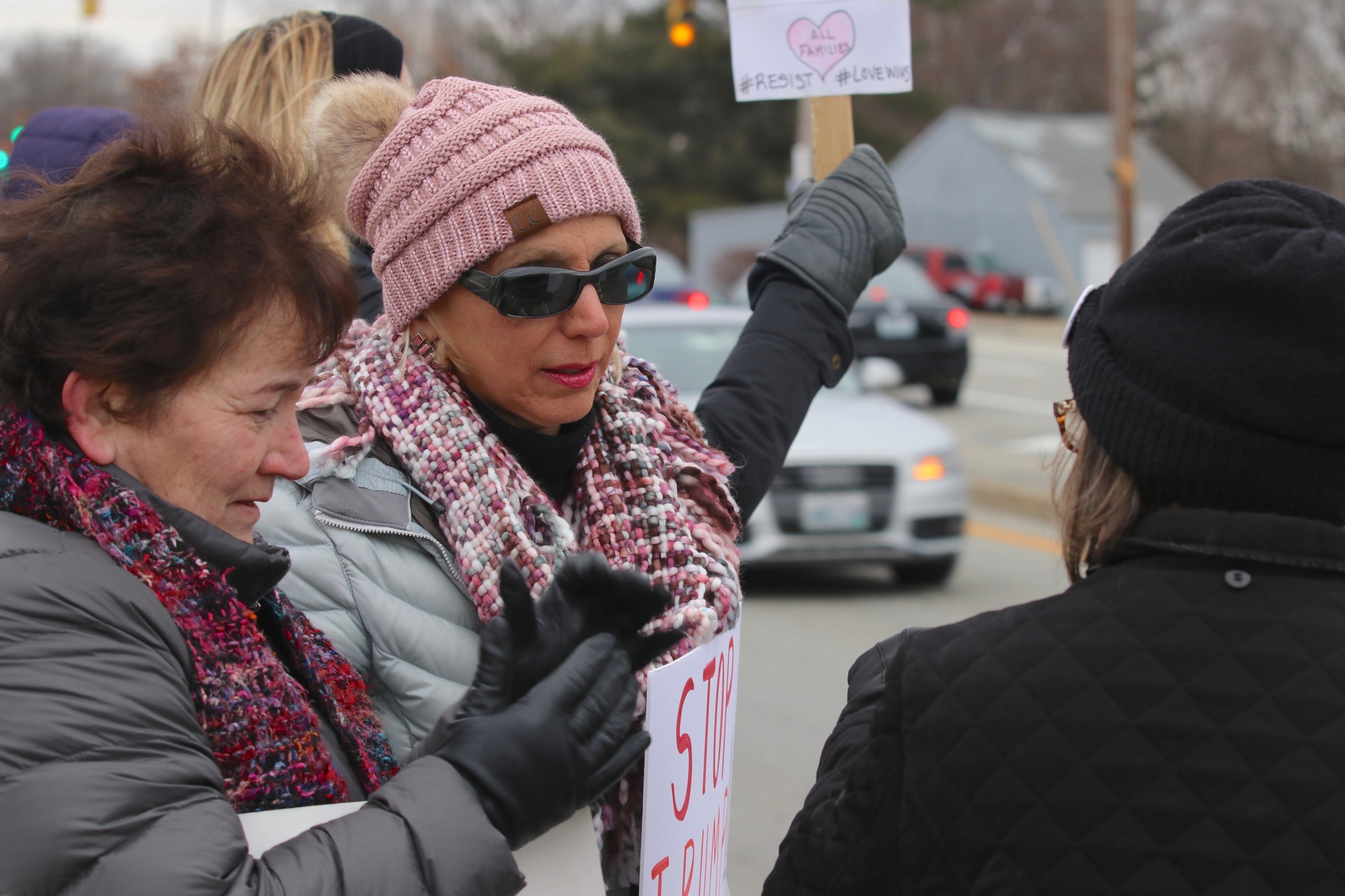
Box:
[905,243,1006,312]
[625,248,715,309]
[847,258,973,408]
[620,300,968,589]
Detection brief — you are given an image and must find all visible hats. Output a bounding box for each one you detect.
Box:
[1061,179,1345,524]
[295,69,642,333]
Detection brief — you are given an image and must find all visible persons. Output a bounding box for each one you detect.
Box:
[246,74,737,896]
[761,182,1345,896]
[197,11,906,517]
[0,109,685,896]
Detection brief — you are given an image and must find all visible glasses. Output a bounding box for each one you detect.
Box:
[1053,398,1090,453]
[456,237,656,319]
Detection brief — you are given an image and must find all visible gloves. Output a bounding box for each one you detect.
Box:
[433,615,653,851]
[746,143,907,323]
[495,551,678,705]
